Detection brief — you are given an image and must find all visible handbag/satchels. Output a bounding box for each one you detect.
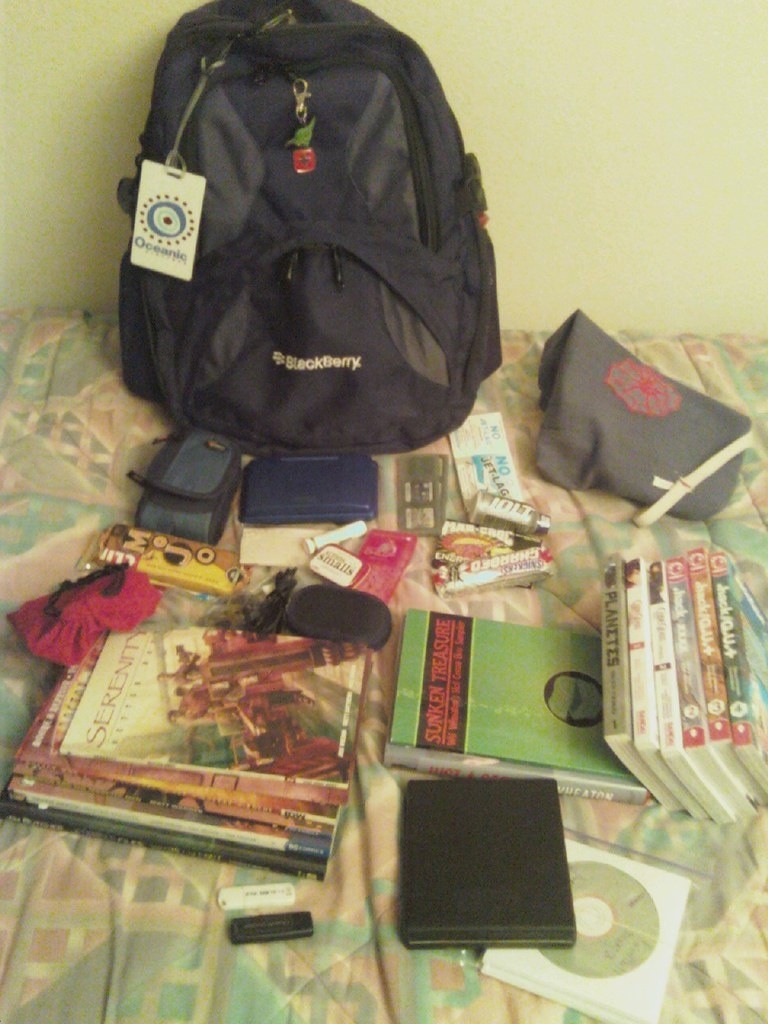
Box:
[5,561,158,667]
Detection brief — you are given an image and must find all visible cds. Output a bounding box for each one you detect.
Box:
[539,861,661,981]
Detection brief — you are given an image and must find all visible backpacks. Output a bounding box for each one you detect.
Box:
[116,1,504,456]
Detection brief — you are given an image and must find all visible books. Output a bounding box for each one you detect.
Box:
[384,548,768,950]
[0,622,373,883]
